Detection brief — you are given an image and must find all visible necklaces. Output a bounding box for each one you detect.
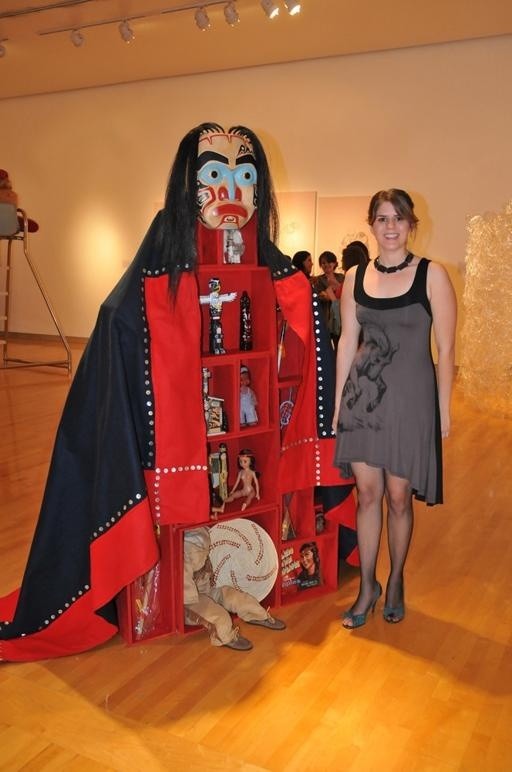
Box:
[374,253,414,273]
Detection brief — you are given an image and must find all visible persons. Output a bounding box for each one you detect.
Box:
[344,239,370,278]
[292,252,313,278]
[297,543,320,586]
[317,252,345,345]
[198,277,238,356]
[214,451,261,515]
[239,365,258,427]
[332,192,458,627]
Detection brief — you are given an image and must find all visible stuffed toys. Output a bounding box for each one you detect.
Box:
[183,528,286,649]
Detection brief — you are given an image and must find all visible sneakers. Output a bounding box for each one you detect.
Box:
[223,635,253,651]
[248,616,286,630]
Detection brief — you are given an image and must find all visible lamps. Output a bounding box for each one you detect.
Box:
[37,0,301,56]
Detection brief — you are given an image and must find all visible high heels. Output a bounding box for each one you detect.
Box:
[383,582,406,623]
[342,584,383,629]
